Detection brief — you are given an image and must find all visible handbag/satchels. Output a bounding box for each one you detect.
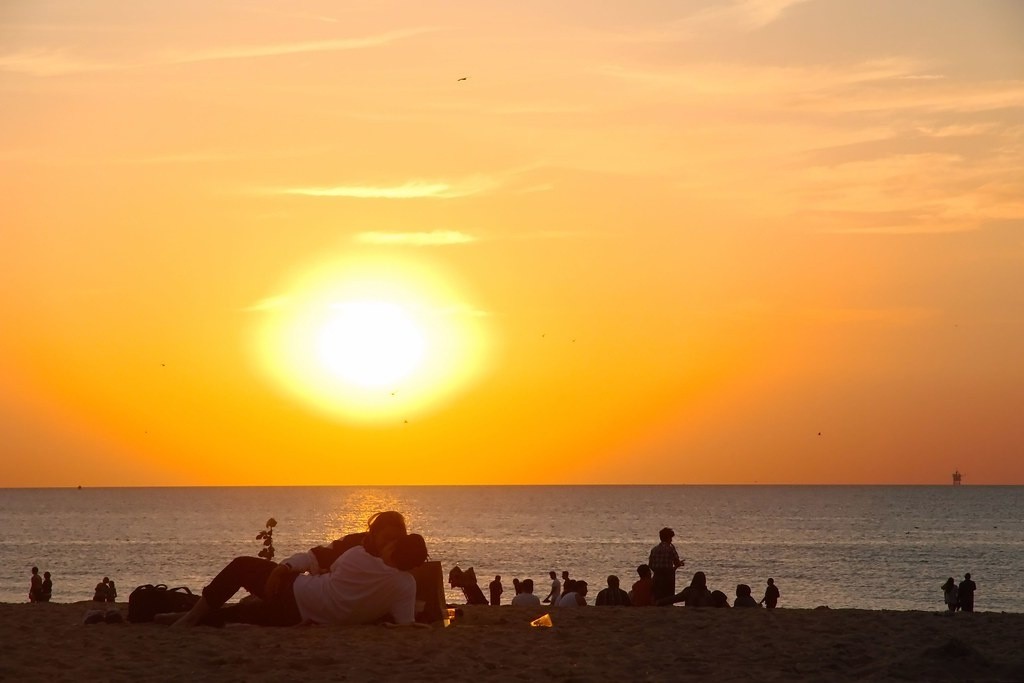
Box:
[124,584,227,629]
[390,553,451,625]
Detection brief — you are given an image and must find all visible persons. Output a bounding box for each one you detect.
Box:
[28,567,42,603]
[511,578,541,608]
[562,571,570,591]
[941,577,959,612]
[649,527,682,606]
[672,571,763,609]
[42,571,52,603]
[93,576,109,602]
[595,575,632,607]
[543,571,561,606]
[554,579,589,606]
[630,563,656,607]
[958,573,977,612]
[760,578,780,608]
[153,511,429,631]
[489,575,503,605]
[107,581,117,603]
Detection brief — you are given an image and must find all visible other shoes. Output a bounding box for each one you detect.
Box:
[82,607,106,625]
[102,605,122,622]
